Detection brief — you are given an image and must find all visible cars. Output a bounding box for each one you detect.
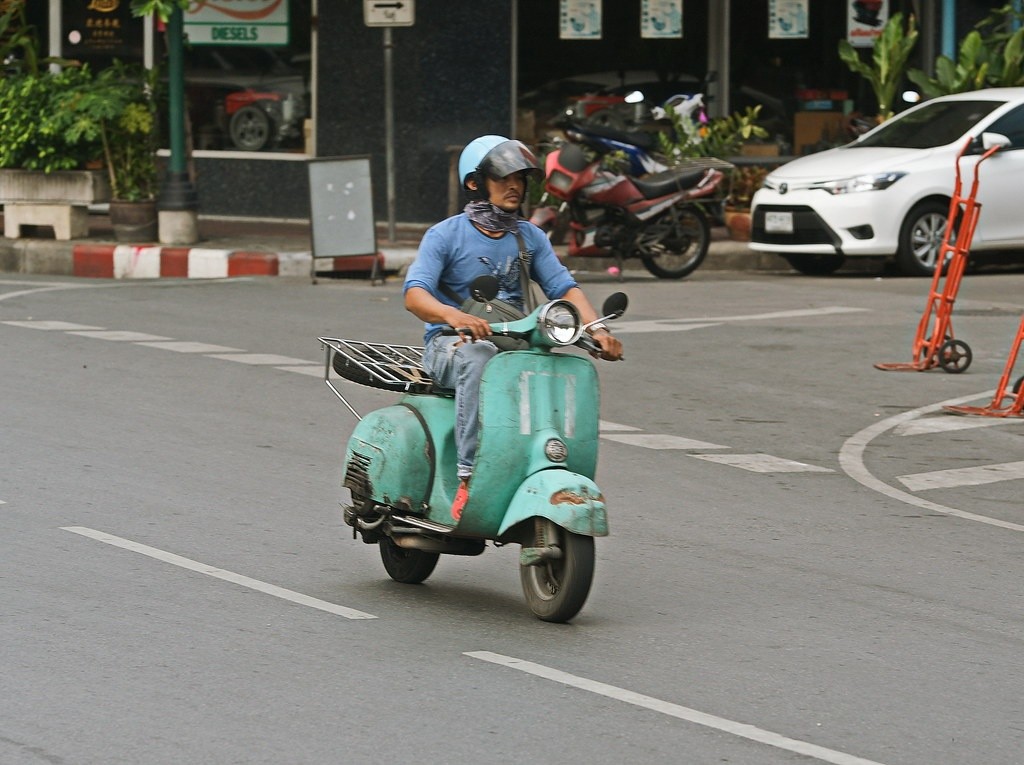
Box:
[145,52,302,150]
[508,60,852,161]
[747,85,1024,277]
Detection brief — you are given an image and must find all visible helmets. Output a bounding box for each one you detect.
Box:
[458,135,531,204]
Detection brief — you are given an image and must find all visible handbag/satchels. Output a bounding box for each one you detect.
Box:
[460,295,533,351]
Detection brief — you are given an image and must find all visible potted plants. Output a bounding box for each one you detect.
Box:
[718,165,770,241]
[43,60,166,242]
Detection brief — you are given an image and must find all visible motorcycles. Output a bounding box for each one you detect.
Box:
[525,103,735,280]
[554,70,728,205]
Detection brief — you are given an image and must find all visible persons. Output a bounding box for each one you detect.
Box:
[401,132,625,524]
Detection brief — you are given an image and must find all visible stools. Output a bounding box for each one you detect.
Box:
[626,162,704,200]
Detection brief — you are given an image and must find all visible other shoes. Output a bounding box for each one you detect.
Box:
[451,482,469,521]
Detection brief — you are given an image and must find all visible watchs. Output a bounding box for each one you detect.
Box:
[584,322,611,335]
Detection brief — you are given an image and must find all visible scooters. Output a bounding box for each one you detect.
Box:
[846,89,923,147]
[315,275,628,626]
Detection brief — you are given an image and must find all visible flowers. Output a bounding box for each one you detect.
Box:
[654,94,718,169]
